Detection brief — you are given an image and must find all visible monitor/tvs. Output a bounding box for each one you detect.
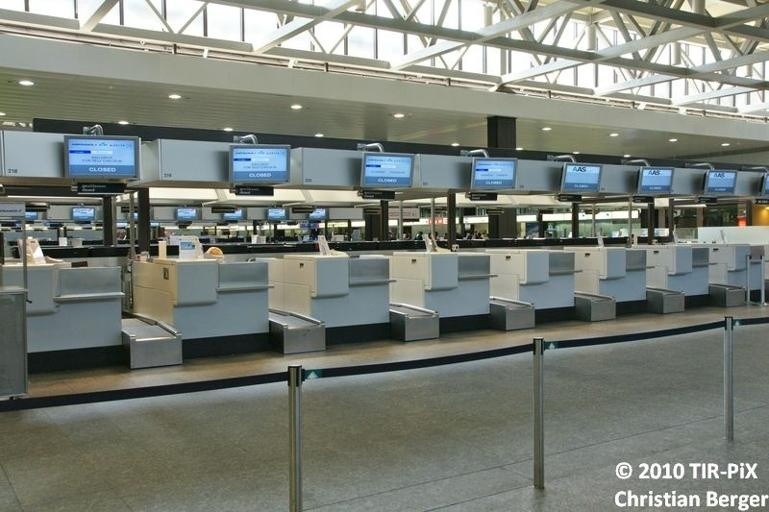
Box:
[228,144,291,185]
[759,171,769,196]
[703,169,738,196]
[360,151,415,189]
[559,162,603,193]
[23,206,330,226]
[61,134,142,182]
[470,157,517,192]
[635,168,675,196]
